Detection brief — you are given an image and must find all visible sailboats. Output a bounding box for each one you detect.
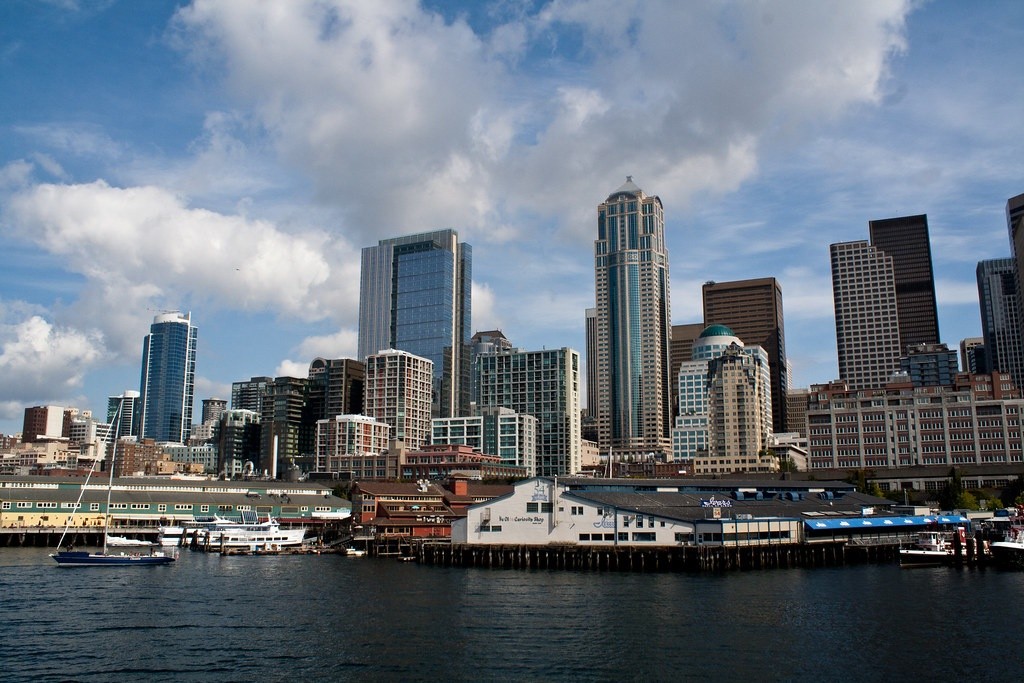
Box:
[49,395,180,567]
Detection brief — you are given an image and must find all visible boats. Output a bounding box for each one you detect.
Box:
[105,532,179,558]
[899,530,992,562]
[990,529,1024,563]
[155,512,369,560]
